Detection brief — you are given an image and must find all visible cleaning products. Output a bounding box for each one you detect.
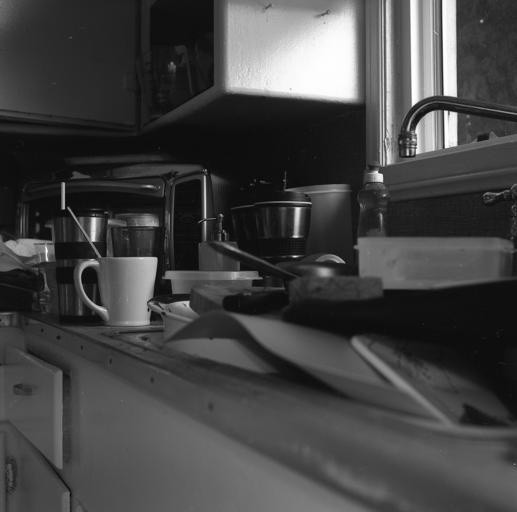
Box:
[356,160,389,237]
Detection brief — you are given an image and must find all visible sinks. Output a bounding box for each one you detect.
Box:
[96,322,516,438]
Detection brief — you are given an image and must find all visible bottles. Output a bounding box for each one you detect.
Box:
[356,167,389,242]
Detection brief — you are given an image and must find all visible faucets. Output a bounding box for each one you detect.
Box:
[396,94,517,160]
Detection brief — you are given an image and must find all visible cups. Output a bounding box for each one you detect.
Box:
[226,192,260,273]
[253,190,312,275]
[70,254,160,328]
[32,240,54,318]
[111,210,162,259]
[53,204,107,326]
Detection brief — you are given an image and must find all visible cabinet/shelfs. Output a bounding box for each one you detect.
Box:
[0,0,141,141]
[0,318,75,512]
[141,0,368,133]
